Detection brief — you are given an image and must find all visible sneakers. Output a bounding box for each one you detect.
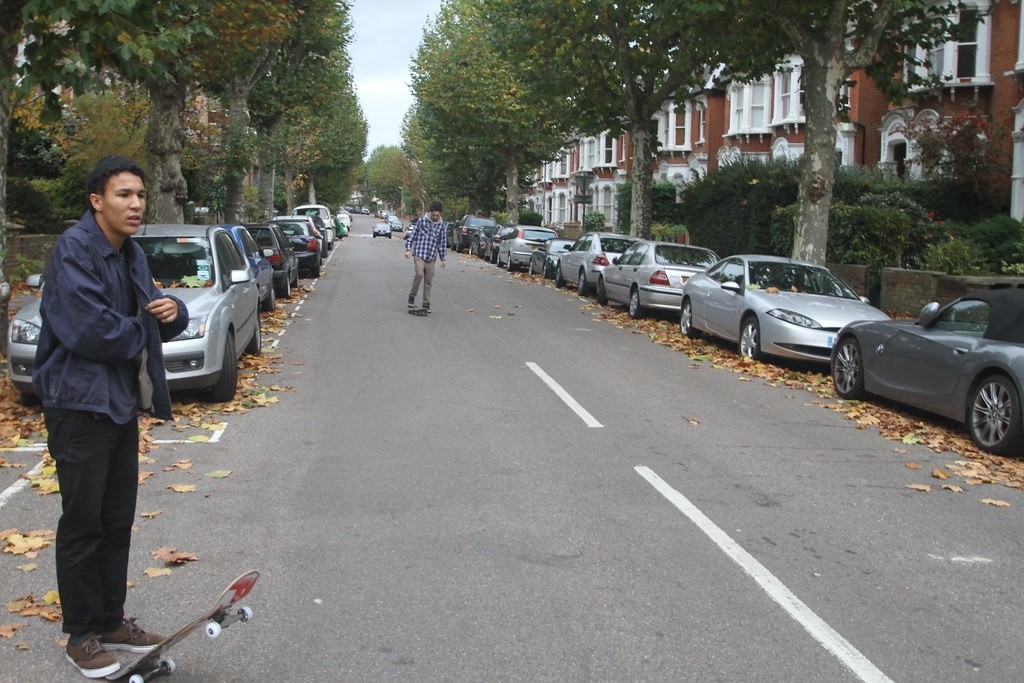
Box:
[65,633,120,678]
[97,618,166,653]
[423,306,431,312]
[408,299,415,306]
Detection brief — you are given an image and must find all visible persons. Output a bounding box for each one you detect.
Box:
[31,155,189,679]
[405,201,448,313]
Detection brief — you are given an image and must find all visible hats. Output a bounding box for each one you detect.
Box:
[430,201,443,212]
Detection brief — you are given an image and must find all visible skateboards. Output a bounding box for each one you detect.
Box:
[105,569,262,683]
[407,305,429,316]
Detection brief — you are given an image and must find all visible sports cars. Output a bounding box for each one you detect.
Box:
[830,285,1023,455]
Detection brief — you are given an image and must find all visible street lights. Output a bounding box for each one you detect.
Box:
[573,166,595,236]
[398,186,404,219]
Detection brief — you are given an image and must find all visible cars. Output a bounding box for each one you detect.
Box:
[372,222,393,239]
[555,231,648,297]
[215,224,276,312]
[678,254,894,370]
[528,238,579,280]
[361,208,370,215]
[6,223,261,403]
[597,240,724,320]
[241,223,300,299]
[402,214,516,264]
[495,224,559,272]
[268,221,323,279]
[0,241,11,358]
[375,208,404,232]
[272,204,361,258]
